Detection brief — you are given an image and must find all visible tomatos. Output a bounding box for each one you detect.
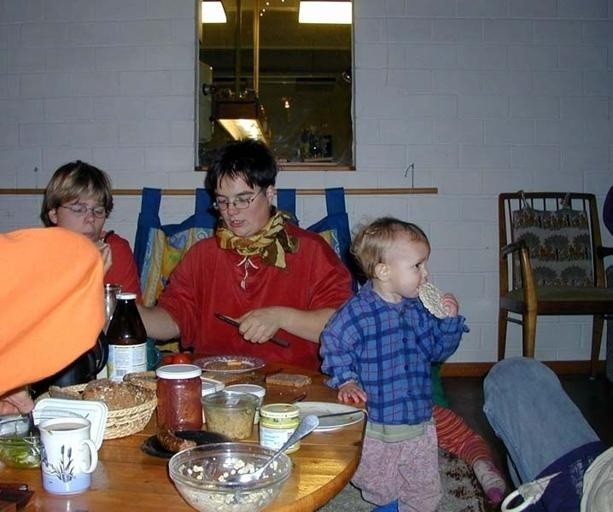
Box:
[174,353,192,364]
[163,355,174,364]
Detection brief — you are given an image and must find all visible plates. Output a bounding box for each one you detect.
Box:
[192,353,268,376]
[139,430,232,460]
[290,401,366,435]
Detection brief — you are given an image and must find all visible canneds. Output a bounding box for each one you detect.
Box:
[260,404,301,454]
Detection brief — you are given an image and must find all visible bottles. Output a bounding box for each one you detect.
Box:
[105,292,149,385]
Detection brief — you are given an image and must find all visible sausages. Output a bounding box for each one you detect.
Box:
[157,429,196,451]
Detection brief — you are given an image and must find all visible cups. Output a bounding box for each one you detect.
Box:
[144,336,161,370]
[36,415,100,497]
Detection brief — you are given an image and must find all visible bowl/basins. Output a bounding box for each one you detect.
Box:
[0,417,43,470]
[167,440,293,512]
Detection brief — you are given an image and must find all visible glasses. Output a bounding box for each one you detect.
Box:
[212,188,263,209]
[60,203,107,218]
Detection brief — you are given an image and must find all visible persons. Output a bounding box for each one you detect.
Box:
[40,158,144,308]
[97,137,355,371]
[316,215,472,512]
[480,356,612,512]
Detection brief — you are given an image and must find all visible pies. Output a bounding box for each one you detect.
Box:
[419,282,449,320]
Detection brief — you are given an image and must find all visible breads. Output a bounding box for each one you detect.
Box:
[83,379,154,411]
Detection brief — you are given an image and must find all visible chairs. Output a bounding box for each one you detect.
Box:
[496,190,613,360]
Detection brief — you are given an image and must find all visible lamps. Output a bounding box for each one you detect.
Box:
[213,0,271,149]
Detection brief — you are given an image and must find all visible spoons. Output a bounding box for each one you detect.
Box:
[218,413,320,493]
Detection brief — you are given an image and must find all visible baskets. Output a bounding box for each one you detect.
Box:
[31,381,158,442]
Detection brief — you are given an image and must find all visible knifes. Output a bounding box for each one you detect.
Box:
[213,311,291,348]
